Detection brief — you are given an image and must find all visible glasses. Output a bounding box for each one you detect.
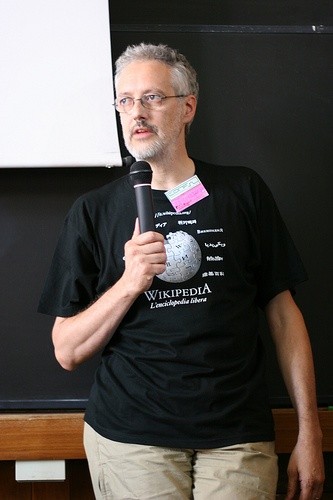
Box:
[112,93,188,113]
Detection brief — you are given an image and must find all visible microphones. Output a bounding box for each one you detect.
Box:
[129,161,156,234]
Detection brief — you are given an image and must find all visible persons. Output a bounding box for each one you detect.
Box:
[52,43,325,500]
